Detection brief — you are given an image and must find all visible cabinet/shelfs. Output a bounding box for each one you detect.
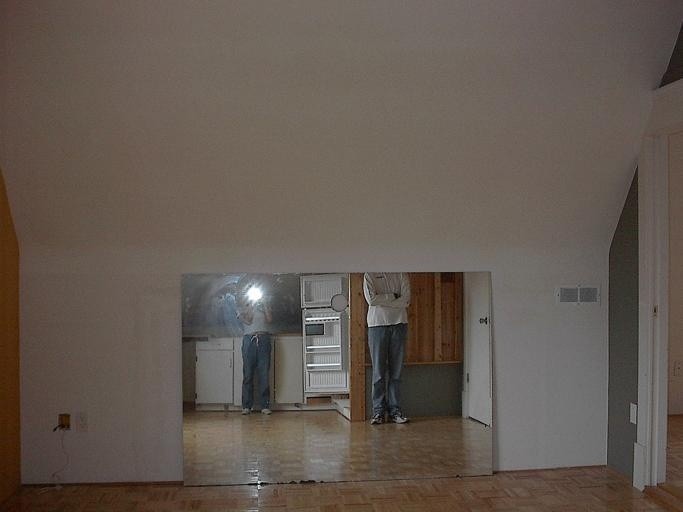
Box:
[191,333,305,413]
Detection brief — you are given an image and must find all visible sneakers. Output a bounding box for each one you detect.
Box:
[370,412,385,424]
[386,414,408,423]
[241,407,273,415]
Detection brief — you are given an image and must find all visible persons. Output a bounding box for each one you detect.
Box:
[364,272,412,424]
[235,297,275,415]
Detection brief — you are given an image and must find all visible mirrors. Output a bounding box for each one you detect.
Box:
[180,269,496,485]
